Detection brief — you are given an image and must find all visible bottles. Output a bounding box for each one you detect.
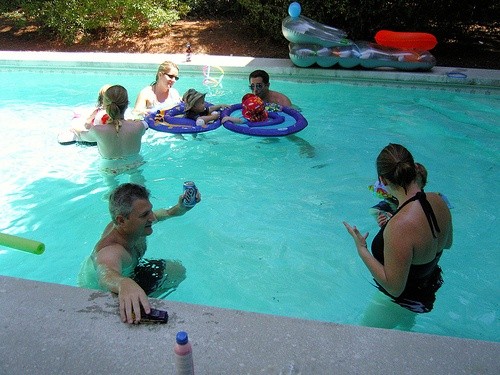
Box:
[186,46,191,62]
[173,331,194,375]
[196,119,205,126]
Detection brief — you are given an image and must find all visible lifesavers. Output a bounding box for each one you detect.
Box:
[0,232,45,255]
[144,101,223,134]
[374,30,439,51]
[220,102,308,138]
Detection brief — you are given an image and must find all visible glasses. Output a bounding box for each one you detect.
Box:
[164,72,179,80]
[249,84,263,90]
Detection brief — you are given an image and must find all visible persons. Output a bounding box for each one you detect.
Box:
[249,69,291,107]
[89,85,146,159]
[221,93,268,124]
[135,61,181,110]
[182,89,231,123]
[85,85,113,130]
[343,144,453,297]
[95,183,201,324]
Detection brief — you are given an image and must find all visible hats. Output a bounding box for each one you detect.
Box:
[242,93,268,122]
[182,88,207,112]
